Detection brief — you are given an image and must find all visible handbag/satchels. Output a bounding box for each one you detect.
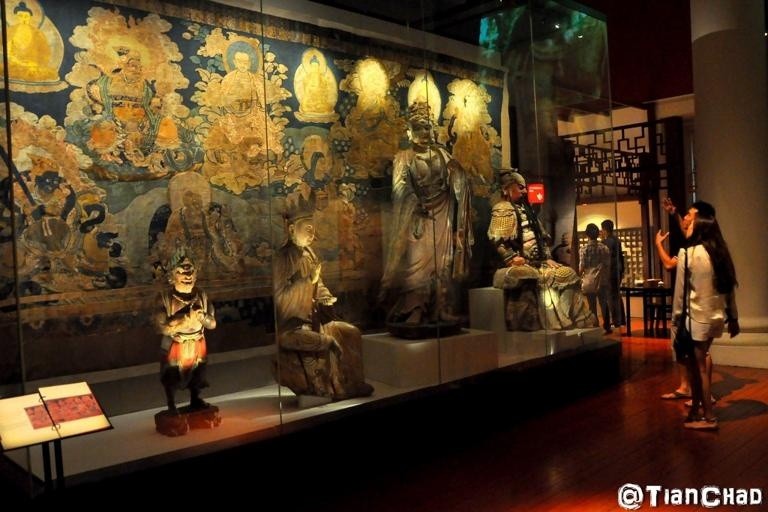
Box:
[579,261,604,295]
[672,325,695,366]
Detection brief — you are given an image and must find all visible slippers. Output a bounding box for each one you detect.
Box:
[660,388,720,430]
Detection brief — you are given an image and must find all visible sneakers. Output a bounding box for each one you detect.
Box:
[591,319,627,336]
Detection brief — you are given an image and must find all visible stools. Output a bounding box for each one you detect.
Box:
[647,302,674,337]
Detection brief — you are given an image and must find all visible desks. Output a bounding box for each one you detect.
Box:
[620,286,671,336]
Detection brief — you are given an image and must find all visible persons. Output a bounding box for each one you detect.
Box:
[579,221,613,334]
[654,193,718,409]
[599,219,627,329]
[668,210,740,432]
[152,245,217,415]
[271,208,370,401]
[486,164,603,332]
[376,98,476,327]
